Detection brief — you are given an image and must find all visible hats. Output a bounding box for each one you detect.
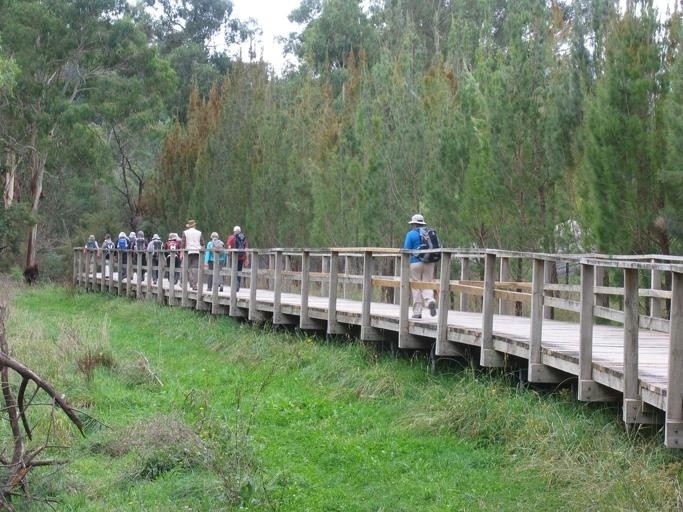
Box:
[89,235,95,240]
[168,233,177,240]
[186,220,197,227]
[152,234,160,240]
[211,232,219,239]
[408,214,426,225]
[105,234,111,240]
[118,231,144,238]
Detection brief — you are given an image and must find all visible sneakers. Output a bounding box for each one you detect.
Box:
[412,315,421,318]
[429,302,436,316]
[191,284,223,291]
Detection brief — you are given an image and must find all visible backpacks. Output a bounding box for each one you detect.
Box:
[414,226,443,263]
[213,239,224,254]
[105,242,114,256]
[154,241,162,254]
[235,234,246,257]
[169,243,178,257]
[87,240,96,253]
[118,239,145,250]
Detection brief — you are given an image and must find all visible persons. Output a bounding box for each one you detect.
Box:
[146,233,165,284]
[83,230,149,281]
[204,232,228,292]
[402,214,436,318]
[179,219,205,290]
[164,232,182,285]
[226,226,250,291]
